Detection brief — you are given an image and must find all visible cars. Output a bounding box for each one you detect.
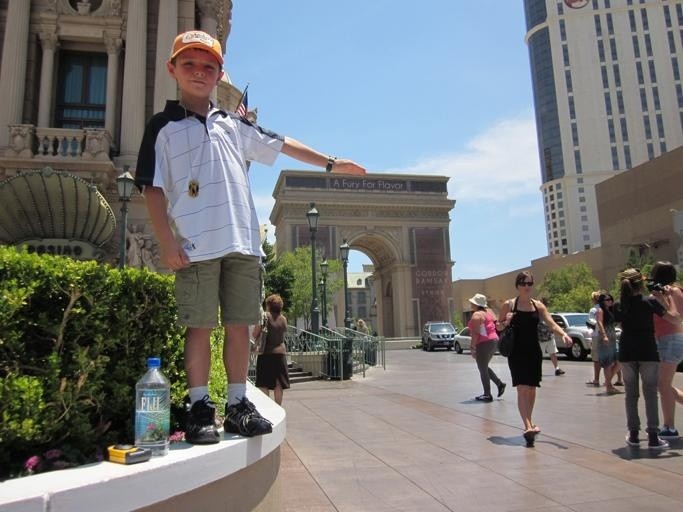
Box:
[453,326,498,355]
[285,328,350,352]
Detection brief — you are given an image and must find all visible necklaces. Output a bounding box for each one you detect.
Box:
[181,102,207,198]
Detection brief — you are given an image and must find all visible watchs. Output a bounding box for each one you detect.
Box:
[324,154,337,173]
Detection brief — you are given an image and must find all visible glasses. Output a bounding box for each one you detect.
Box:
[519,282,533,286]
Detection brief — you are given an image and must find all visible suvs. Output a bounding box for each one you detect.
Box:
[421,321,458,351]
[545,312,622,361]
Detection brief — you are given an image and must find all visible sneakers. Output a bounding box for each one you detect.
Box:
[475,395,493,402]
[224,397,272,437]
[185,395,219,444]
[556,370,564,375]
[627,426,678,448]
[497,384,506,396]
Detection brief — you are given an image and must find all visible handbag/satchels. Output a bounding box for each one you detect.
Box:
[498,327,515,356]
[254,330,267,355]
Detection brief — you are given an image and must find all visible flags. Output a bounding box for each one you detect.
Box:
[234,93,247,118]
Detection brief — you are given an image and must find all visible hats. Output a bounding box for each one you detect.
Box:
[169,30,223,67]
[469,294,487,307]
[621,269,647,281]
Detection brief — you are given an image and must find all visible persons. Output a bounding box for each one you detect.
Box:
[538,317,565,376]
[357,319,367,334]
[497,272,572,445]
[253,293,290,407]
[133,31,368,446]
[586,262,682,449]
[467,294,505,403]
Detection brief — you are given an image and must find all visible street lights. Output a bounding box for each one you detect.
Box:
[316,277,327,342]
[305,202,320,348]
[320,257,329,337]
[339,239,351,355]
[115,165,136,269]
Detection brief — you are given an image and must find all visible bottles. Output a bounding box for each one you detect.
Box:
[133,357,169,453]
